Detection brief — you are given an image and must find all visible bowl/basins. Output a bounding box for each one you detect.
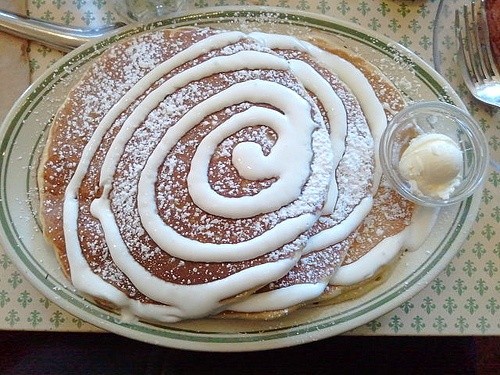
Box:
[380,101,489,207]
[435,1,499,172]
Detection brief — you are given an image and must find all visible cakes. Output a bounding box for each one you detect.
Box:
[36,26,443,327]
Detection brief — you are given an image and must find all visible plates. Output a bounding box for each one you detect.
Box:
[1,7,483,352]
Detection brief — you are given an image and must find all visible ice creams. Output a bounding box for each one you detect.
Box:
[399,133,463,200]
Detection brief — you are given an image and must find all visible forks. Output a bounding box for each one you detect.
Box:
[452,0,500,108]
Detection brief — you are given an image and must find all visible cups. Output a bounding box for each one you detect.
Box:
[105,0,188,24]
